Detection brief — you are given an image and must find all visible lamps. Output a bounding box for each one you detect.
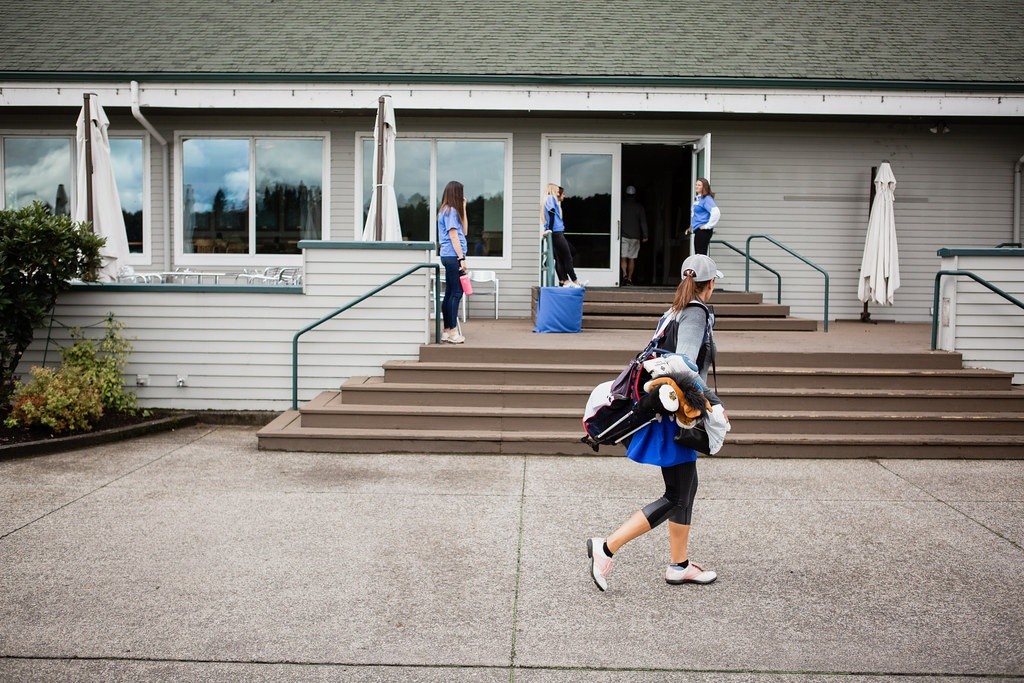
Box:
[929,126,950,134]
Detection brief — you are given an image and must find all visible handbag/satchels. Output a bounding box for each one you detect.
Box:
[581,339,731,455]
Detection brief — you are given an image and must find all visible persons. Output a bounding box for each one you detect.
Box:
[437,180,469,344]
[692,178,721,256]
[587,253,725,593]
[473,230,490,256]
[620,186,648,285]
[543,184,577,287]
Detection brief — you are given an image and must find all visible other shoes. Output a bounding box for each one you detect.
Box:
[559,282,564,286]
[626,280,633,285]
[578,278,589,288]
[563,282,581,288]
[622,276,628,285]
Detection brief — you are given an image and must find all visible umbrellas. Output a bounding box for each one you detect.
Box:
[363,95,403,243]
[184,185,196,253]
[859,160,900,316]
[73,91,131,282]
[55,184,70,215]
[298,187,322,240]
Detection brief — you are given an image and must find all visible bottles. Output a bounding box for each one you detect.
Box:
[459,269,473,295]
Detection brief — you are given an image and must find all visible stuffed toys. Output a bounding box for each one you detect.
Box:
[644,375,712,429]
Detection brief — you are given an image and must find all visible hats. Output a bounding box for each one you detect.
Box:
[626,186,636,194]
[681,254,724,282]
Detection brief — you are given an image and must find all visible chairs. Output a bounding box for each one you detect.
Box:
[431,266,499,321]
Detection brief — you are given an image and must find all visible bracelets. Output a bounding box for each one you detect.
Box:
[457,258,465,262]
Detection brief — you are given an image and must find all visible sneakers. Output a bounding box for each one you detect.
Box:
[441,330,465,343]
[588,536,613,592]
[665,561,717,584]
